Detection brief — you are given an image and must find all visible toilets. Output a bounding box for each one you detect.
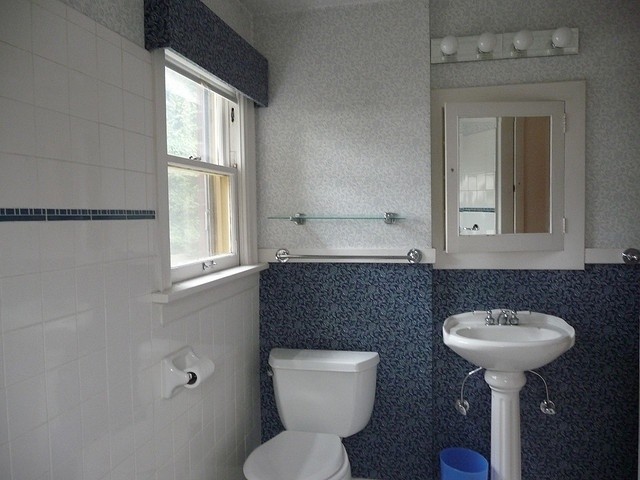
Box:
[242,347,380,480]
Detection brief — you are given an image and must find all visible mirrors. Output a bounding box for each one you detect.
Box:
[443,102,564,252]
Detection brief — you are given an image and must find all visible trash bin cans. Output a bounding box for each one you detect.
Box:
[440,447,489,480]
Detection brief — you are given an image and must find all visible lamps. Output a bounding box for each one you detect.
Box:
[550,26,572,51]
[476,31,494,54]
[439,37,458,56]
[513,29,533,52]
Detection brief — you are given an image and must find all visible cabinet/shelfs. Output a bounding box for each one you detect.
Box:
[269,211,423,268]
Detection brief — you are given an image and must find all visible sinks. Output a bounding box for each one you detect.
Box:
[442,309,575,370]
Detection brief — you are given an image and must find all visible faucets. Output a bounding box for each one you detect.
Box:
[461,224,480,233]
[497,310,511,326]
